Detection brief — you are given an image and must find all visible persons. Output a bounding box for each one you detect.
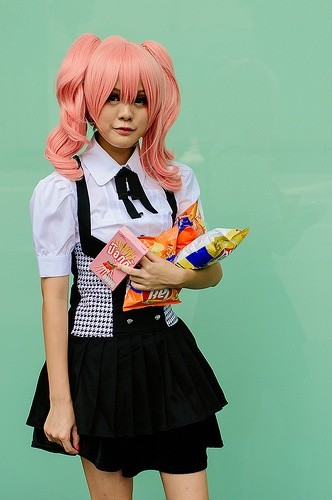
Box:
[24,35,229,500]
